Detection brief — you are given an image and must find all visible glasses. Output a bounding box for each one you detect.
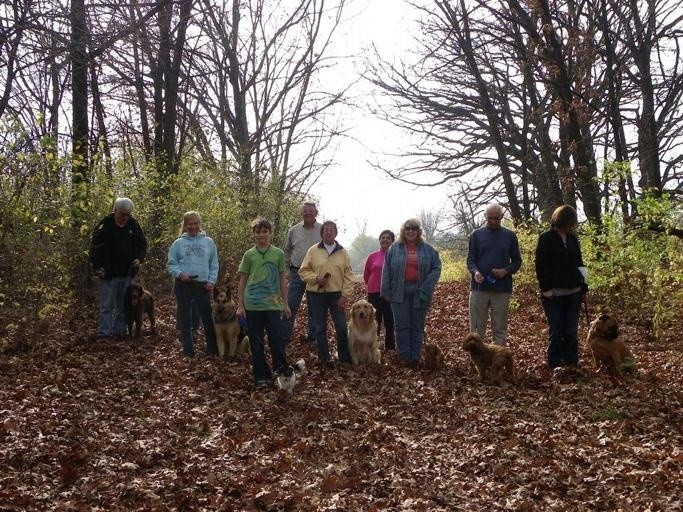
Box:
[116,210,130,217]
[404,226,418,232]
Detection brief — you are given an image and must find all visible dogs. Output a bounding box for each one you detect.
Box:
[586,314,635,371]
[462,332,515,386]
[212,281,250,361]
[424,341,444,371]
[273,358,308,394]
[347,298,382,373]
[123,283,156,339]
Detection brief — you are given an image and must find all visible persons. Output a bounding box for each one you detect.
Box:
[166,210,219,360]
[234,216,291,390]
[296,219,354,366]
[378,217,441,365]
[362,230,395,354]
[466,202,521,346]
[280,201,322,343]
[86,196,147,344]
[534,204,589,368]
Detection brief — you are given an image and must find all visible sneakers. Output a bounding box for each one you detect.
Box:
[206,350,217,359]
[101,333,114,343]
[116,331,126,341]
[182,350,196,363]
[254,379,272,390]
